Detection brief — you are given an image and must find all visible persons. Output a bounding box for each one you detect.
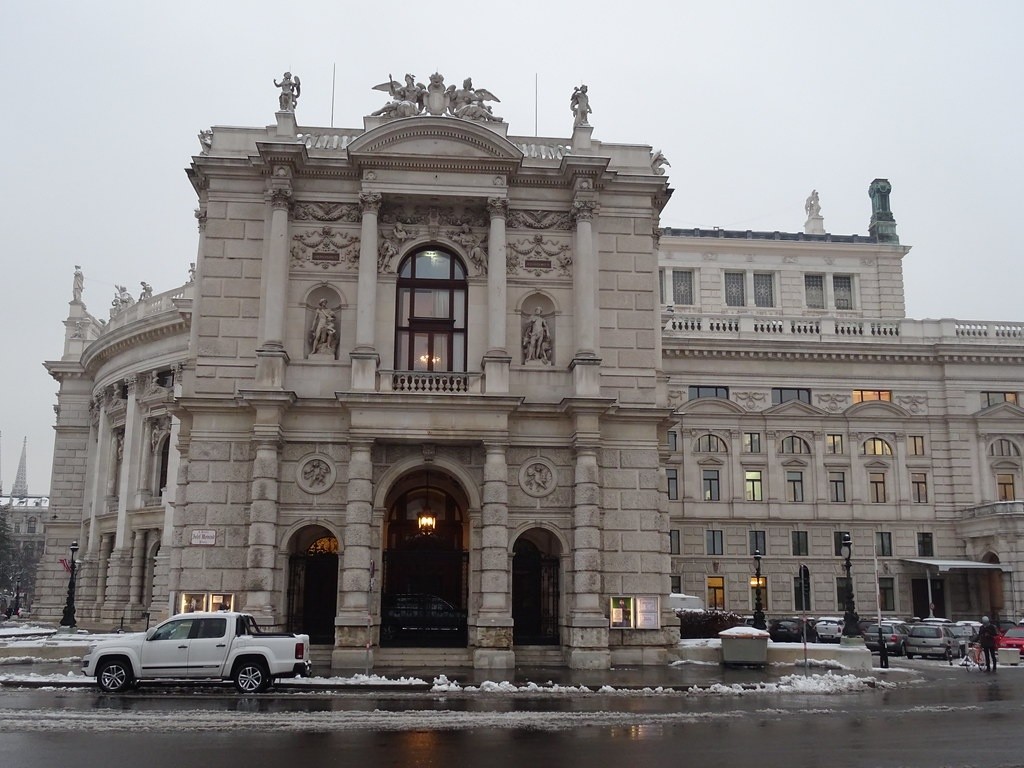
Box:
[6,605,24,620]
[445,78,504,123]
[972,616,999,673]
[525,306,552,361]
[70,263,196,337]
[813,192,821,216]
[93,369,159,419]
[570,85,593,124]
[274,72,301,112]
[370,72,425,118]
[310,298,336,353]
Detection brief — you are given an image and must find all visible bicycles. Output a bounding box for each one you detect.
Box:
[966,639,988,672]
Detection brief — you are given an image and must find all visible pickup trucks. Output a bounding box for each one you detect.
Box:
[80,609,314,691]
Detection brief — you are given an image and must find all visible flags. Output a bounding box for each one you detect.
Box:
[60,559,72,572]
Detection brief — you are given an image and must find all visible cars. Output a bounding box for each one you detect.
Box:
[814,617,847,643]
[380,592,467,646]
[906,623,962,658]
[736,616,768,632]
[999,625,1024,655]
[869,615,1016,650]
[769,617,819,644]
[864,622,910,656]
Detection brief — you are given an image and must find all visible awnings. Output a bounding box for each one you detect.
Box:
[902,559,1012,572]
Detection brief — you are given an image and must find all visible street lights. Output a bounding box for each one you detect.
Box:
[59,541,80,626]
[753,550,768,632]
[13,577,21,615]
[841,533,866,647]
